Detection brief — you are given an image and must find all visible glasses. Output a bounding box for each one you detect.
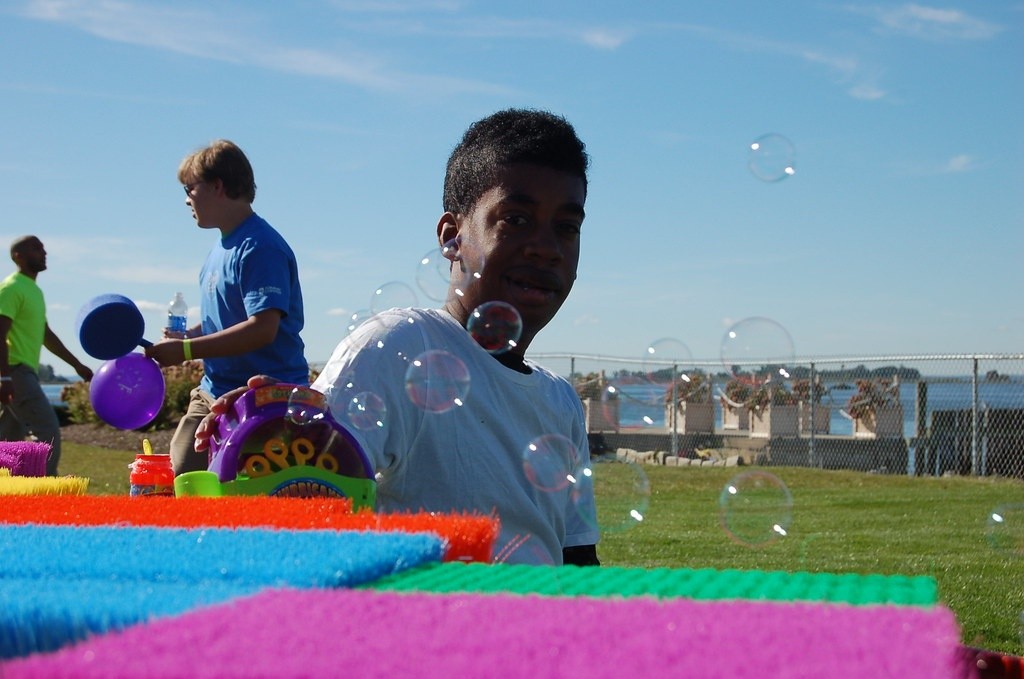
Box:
[184,181,208,197]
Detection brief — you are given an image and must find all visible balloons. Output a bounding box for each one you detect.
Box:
[89,351,165,428]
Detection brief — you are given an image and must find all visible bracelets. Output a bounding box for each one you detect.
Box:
[181,337,193,362]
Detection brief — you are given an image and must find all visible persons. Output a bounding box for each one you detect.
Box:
[192,106,603,566]
[139,136,310,479]
[0,235,95,474]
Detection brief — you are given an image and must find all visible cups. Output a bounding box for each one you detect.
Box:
[130,454,175,497]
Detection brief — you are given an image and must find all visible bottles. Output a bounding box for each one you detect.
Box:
[167,292,188,339]
